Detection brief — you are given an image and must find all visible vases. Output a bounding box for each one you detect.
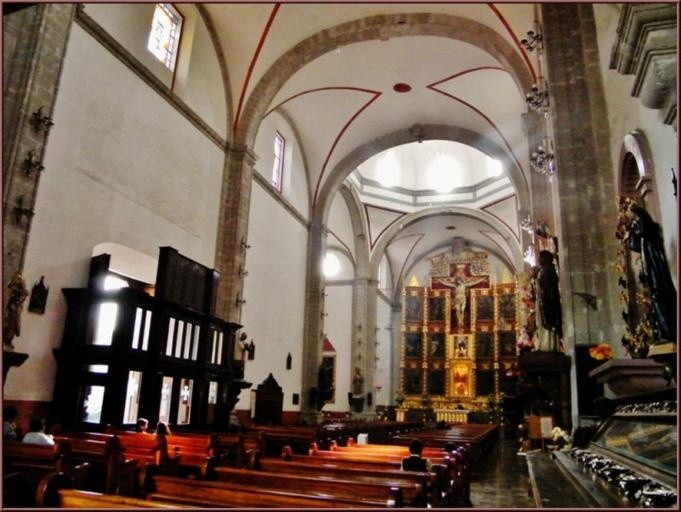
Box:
[588,359,670,399]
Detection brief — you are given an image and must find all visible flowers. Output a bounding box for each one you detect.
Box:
[589,200,661,362]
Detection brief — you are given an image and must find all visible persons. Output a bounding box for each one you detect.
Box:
[445,442,457,452]
[535,250,562,351]
[437,419,450,429]
[130,418,148,435]
[3,405,18,440]
[154,423,173,435]
[437,275,486,327]
[528,266,539,302]
[400,438,432,473]
[21,415,56,446]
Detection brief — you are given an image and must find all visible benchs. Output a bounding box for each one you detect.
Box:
[2,420,501,512]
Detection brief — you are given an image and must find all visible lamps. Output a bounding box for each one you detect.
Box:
[526,80,549,113]
[14,106,54,224]
[529,137,554,176]
[529,417,553,454]
[520,23,544,54]
